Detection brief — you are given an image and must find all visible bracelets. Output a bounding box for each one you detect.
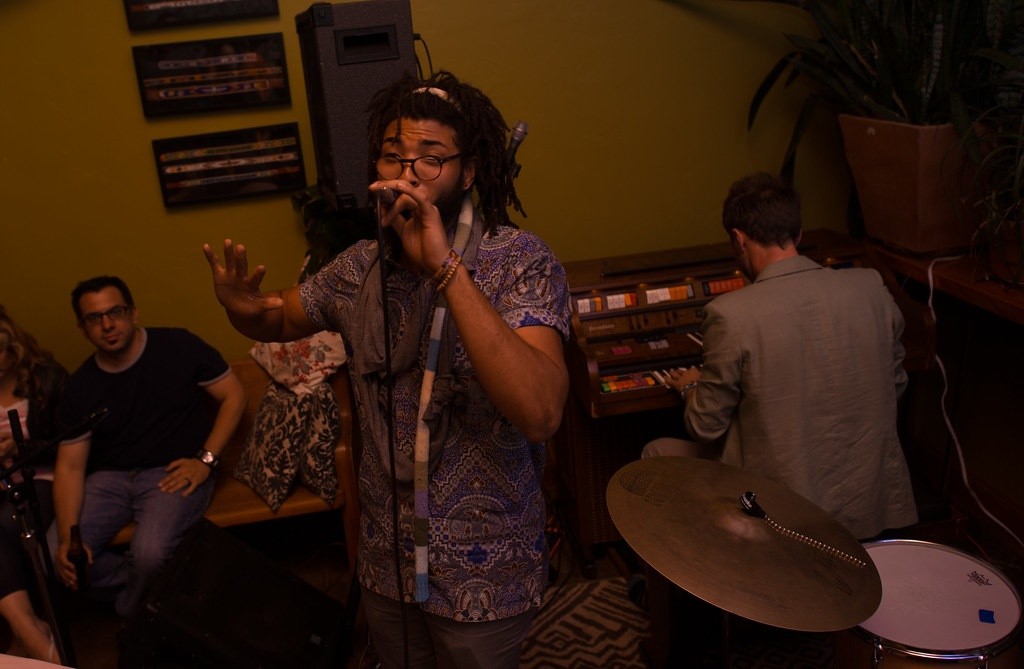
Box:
[681,382,692,398]
[432,249,463,291]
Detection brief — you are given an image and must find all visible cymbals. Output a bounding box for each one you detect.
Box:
[605,455,882,634]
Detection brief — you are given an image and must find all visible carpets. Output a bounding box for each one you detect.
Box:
[520,574,837,669]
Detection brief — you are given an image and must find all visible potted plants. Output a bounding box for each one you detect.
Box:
[748,0,1024,286]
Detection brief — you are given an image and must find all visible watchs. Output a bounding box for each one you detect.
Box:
[197,448,220,469]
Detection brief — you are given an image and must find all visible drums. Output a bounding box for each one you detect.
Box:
[833,539,1024,669]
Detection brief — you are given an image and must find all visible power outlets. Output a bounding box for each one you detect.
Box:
[950,357,971,379]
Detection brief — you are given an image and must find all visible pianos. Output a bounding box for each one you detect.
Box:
[552,225,940,569]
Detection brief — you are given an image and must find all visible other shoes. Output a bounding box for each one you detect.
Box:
[637,639,712,669]
[116,619,175,669]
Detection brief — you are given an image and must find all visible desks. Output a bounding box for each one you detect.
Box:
[865,238,1024,326]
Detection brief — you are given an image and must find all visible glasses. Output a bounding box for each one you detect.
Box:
[369,152,477,181]
[80,304,133,325]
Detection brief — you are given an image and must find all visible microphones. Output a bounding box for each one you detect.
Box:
[380,187,400,208]
[504,120,528,173]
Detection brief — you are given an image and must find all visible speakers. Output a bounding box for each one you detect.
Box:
[294,0,420,214]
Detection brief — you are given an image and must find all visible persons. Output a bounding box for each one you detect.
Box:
[1,309,85,669]
[44,275,248,617]
[639,169,920,541]
[200,70,572,669]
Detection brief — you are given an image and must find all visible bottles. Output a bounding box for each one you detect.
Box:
[67,525,89,581]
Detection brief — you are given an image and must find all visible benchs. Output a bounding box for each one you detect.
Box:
[108,361,367,625]
